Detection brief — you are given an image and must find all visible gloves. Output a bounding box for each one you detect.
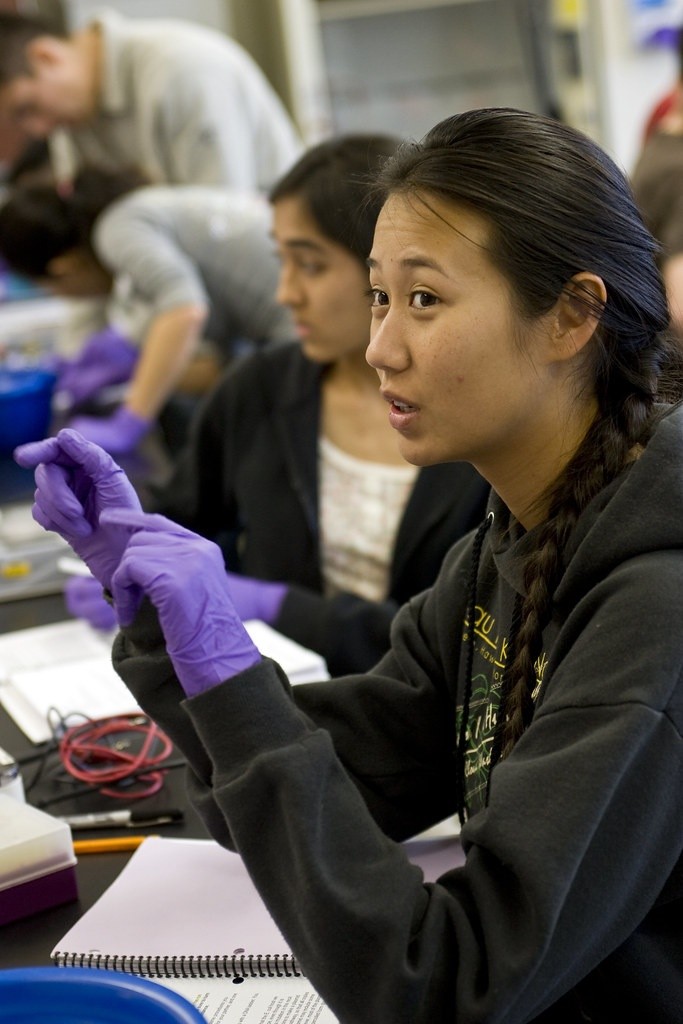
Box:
[62,408,153,455]
[64,576,117,633]
[97,510,261,700]
[15,427,144,595]
[58,323,137,409]
[230,571,288,624]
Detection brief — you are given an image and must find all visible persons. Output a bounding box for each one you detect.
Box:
[0,9,308,202]
[0,166,303,459]
[11,110,683,1024]
[63,138,491,677]
[630,33,682,334]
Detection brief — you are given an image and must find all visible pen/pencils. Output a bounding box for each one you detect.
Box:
[55,809,189,830]
[71,836,156,855]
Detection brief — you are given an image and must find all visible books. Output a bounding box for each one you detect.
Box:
[50,834,345,1024]
[0,608,330,742]
